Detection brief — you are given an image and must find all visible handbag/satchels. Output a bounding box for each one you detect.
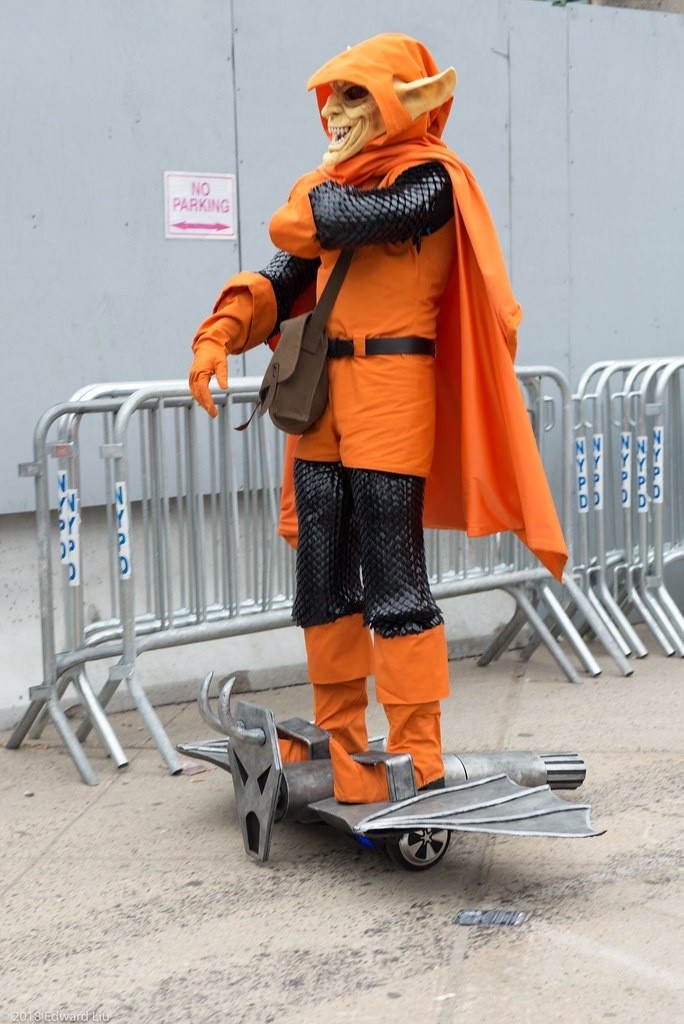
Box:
[234,174,385,435]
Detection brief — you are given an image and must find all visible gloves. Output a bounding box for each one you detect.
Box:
[189,287,253,418]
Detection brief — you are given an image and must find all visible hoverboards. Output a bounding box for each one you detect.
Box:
[170,672,612,873]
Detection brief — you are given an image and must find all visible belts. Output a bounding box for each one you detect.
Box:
[325,336,434,358]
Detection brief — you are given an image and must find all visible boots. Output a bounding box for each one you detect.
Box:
[329,623,451,802]
[278,613,373,763]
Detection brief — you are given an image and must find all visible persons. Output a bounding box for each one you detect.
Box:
[189,33,567,804]
